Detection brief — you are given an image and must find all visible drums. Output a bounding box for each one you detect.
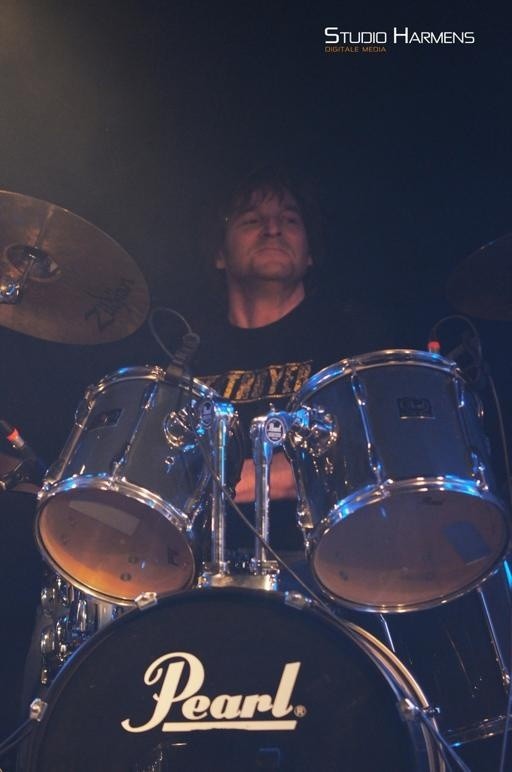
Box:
[40,558,281,694]
[196,561,278,591]
[278,556,512,746]
[25,586,446,771]
[281,349,512,615]
[31,364,247,610]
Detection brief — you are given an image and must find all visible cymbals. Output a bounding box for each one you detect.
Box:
[0,189,151,345]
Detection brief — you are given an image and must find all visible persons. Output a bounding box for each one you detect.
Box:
[0,170,397,654]
